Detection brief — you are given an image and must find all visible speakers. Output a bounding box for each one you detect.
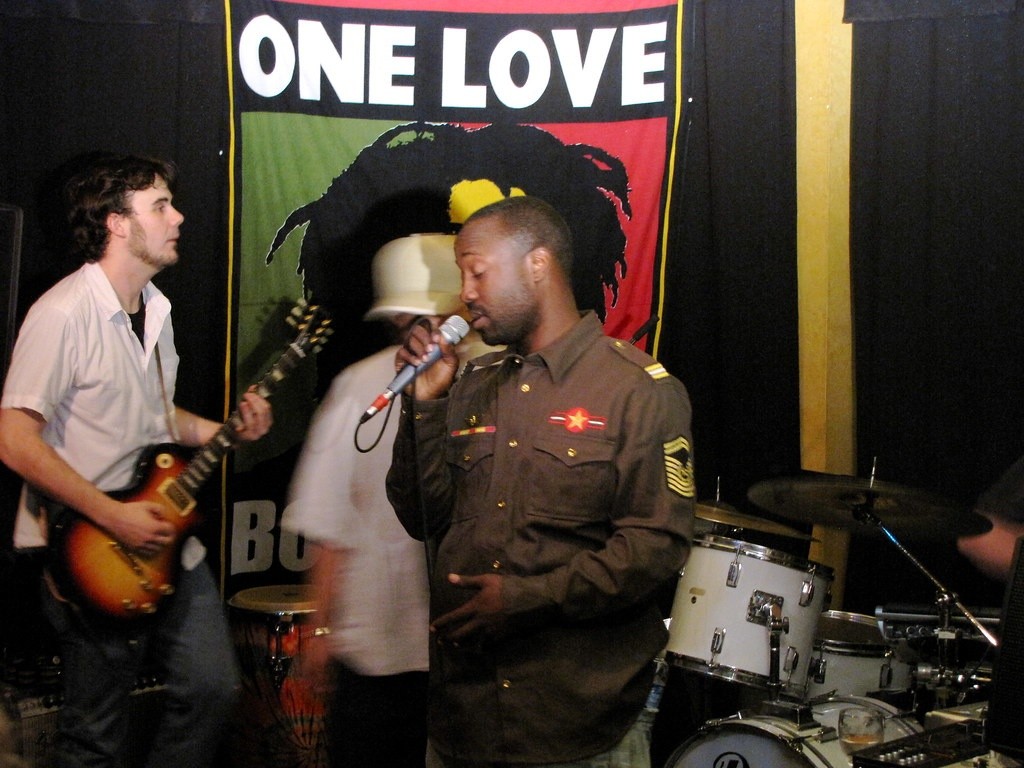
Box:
[0,672,177,768]
[982,537,1024,758]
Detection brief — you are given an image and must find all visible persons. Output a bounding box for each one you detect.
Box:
[0,151,273,768]
[279,196,697,768]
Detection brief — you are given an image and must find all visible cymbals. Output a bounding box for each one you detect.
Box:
[744,473,996,547]
[691,500,820,543]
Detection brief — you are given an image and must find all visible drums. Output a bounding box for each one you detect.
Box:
[664,696,930,768]
[760,608,910,721]
[228,584,334,768]
[655,533,836,700]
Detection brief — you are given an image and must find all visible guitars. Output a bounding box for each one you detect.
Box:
[53,299,335,627]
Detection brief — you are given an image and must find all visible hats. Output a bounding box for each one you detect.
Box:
[365,237,466,322]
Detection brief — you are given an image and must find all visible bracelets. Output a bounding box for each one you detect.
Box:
[297,626,332,637]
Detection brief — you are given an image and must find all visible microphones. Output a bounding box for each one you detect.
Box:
[359,315,470,424]
